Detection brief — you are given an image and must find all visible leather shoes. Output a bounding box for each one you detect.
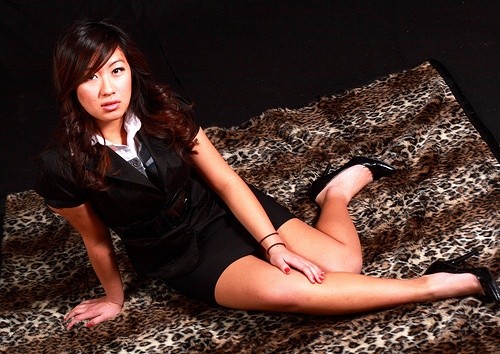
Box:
[423,249,500,305]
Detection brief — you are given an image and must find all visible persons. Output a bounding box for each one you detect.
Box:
[40,21,500,330]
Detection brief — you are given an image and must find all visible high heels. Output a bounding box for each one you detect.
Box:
[310,156,396,202]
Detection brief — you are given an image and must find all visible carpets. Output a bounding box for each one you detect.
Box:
[0,57,500,354]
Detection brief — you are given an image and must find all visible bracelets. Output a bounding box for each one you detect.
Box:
[259,232,279,245]
[267,243,286,255]
